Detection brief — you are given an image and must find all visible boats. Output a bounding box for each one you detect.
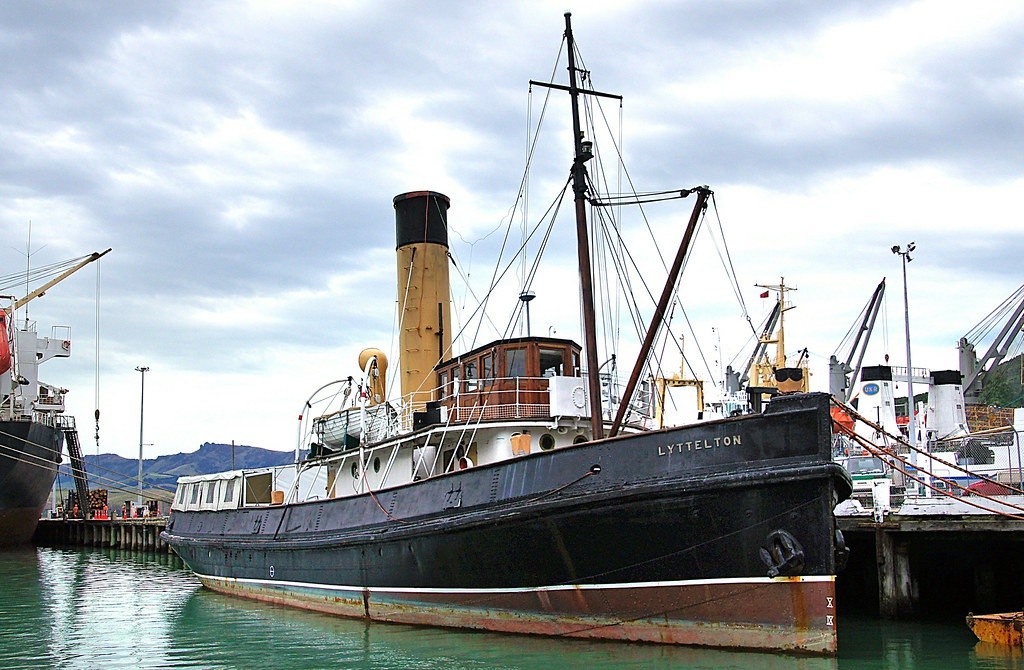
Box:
[160,11,843,658]
[0,294,72,550]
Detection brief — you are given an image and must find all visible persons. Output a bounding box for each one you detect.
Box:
[73,504,78,518]
[122,505,126,518]
[102,504,108,510]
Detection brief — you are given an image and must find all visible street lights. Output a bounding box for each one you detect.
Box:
[133,365,149,505]
[517,291,537,337]
[959,424,975,466]
[891,240,918,494]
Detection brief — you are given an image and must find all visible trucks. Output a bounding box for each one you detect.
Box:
[843,455,906,506]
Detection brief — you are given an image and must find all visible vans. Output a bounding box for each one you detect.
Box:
[932,479,961,496]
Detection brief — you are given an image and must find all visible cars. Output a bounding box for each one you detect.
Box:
[962,471,1021,496]
